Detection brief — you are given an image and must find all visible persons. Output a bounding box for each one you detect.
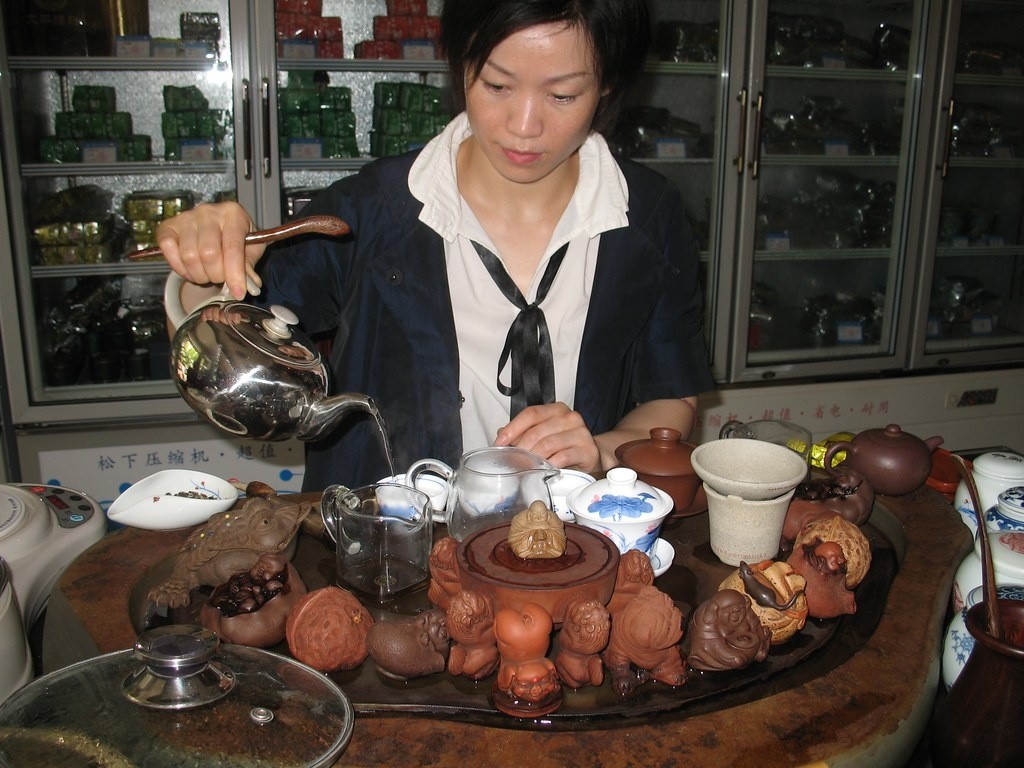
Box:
[155,0,720,496]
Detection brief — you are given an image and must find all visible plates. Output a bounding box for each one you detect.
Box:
[650,538,675,577]
[691,438,807,500]
[107,469,238,531]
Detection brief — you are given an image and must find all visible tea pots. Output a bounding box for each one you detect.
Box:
[164,270,378,443]
[824,423,944,497]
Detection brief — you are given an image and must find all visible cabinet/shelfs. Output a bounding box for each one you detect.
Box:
[0,0,1024,426]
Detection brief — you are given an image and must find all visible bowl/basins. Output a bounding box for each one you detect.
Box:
[521,469,597,523]
[458,466,519,518]
[375,474,449,521]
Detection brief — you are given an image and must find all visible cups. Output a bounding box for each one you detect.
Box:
[615,427,702,512]
[320,483,433,605]
[704,482,794,568]
[566,467,674,561]
[405,446,561,542]
[719,419,812,483]
